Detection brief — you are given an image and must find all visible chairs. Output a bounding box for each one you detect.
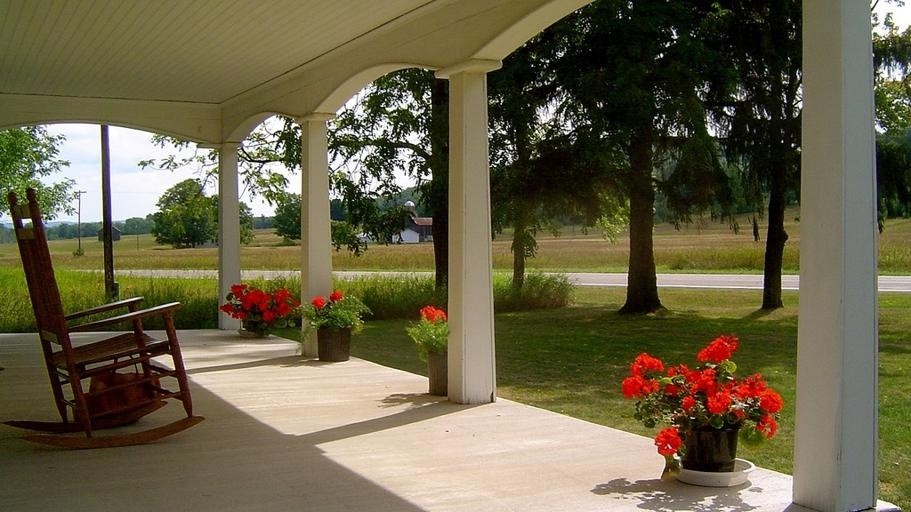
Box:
[0,188,206,449]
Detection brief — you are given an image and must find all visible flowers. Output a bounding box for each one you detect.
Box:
[287,290,375,354]
[219,284,300,336]
[620,332,783,481]
[405,304,448,363]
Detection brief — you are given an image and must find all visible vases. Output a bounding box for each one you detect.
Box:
[674,425,755,487]
[427,348,447,396]
[237,328,264,339]
[318,325,350,362]
